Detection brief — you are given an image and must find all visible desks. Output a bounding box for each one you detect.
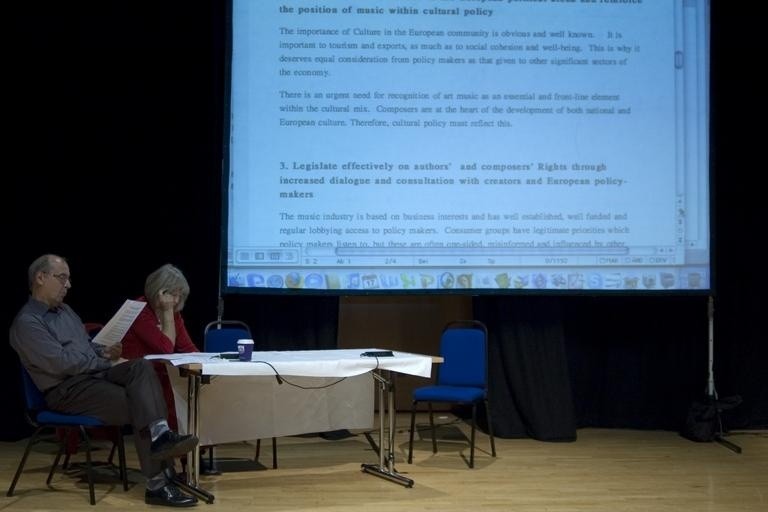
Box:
[145,348,444,503]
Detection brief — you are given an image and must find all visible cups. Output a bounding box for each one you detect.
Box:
[237,339,255,361]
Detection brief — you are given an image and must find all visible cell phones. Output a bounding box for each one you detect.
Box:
[366,352,393,357]
[220,354,239,360]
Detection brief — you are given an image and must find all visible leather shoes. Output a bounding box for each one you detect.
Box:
[144,483,199,507]
[149,431,200,461]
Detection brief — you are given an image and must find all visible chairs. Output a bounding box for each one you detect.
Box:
[56,323,134,469]
[6,362,127,505]
[203,321,277,475]
[407,320,495,469]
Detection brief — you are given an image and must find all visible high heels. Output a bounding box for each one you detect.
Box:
[180,452,220,477]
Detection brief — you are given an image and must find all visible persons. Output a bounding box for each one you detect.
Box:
[9,254,198,506]
[121,263,218,474]
